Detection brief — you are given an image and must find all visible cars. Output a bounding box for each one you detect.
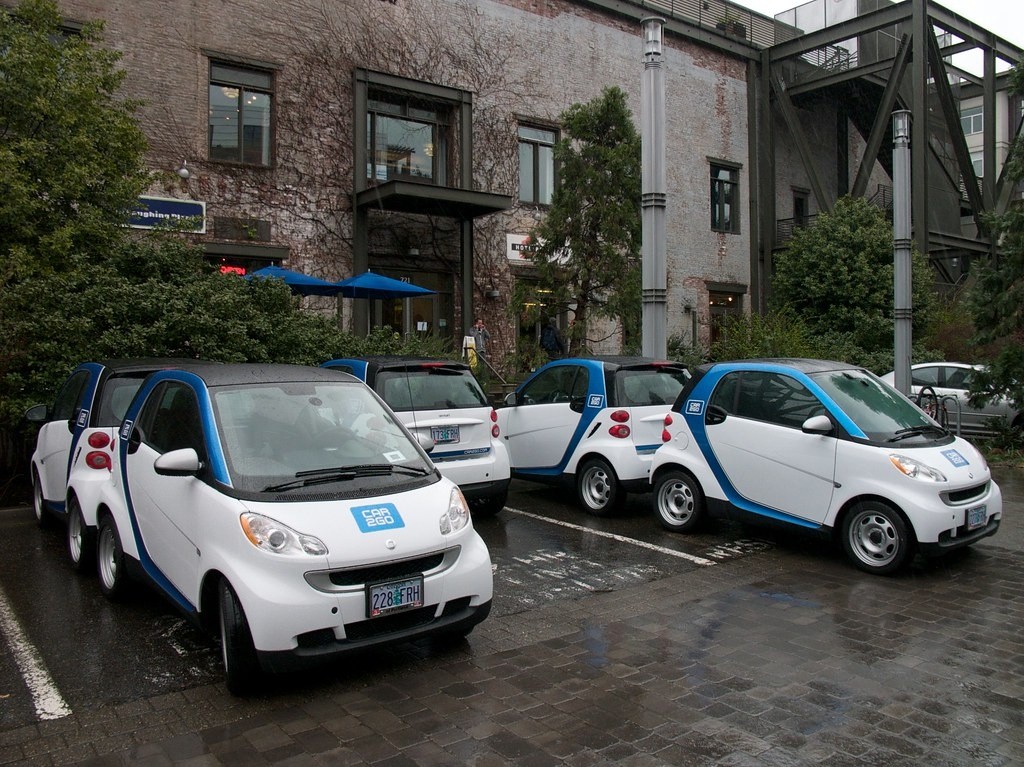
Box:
[494,355,706,520]
[877,363,1024,448]
[648,356,1003,578]
[23,358,223,574]
[317,359,512,516]
[96,361,494,690]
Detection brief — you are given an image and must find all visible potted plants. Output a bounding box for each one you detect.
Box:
[715,10,747,37]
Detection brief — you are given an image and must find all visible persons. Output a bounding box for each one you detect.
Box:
[469,317,490,362]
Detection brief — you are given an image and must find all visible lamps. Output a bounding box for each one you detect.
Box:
[177,159,190,179]
[523,205,541,220]
[484,290,500,298]
[222,86,239,98]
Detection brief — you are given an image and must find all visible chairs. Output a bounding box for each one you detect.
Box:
[286,406,337,444]
[169,390,200,447]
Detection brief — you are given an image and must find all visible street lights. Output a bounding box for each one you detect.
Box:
[639,15,670,361]
[892,109,913,398]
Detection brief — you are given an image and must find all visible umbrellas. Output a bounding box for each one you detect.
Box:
[338,269,439,340]
[242,258,348,311]
